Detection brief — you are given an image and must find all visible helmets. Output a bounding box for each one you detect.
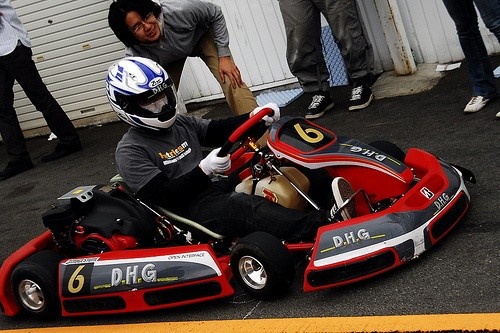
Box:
[105,55,178,131]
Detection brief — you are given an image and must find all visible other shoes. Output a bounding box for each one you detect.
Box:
[0,156,34,181]
[41,143,83,163]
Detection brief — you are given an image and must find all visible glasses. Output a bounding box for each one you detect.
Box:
[130,12,153,34]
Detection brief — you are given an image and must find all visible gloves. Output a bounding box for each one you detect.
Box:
[249,102,281,126]
[198,146,231,178]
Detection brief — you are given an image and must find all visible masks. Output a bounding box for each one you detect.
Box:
[137,92,168,114]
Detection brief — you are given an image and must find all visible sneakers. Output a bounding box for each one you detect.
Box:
[325,176,355,224]
[304,92,334,118]
[464,96,491,113]
[348,81,373,111]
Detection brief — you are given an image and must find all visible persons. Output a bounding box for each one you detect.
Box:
[0,0,82,179]
[278,0,374,119]
[106,57,354,242]
[442,0,500,112]
[108,0,260,115]
